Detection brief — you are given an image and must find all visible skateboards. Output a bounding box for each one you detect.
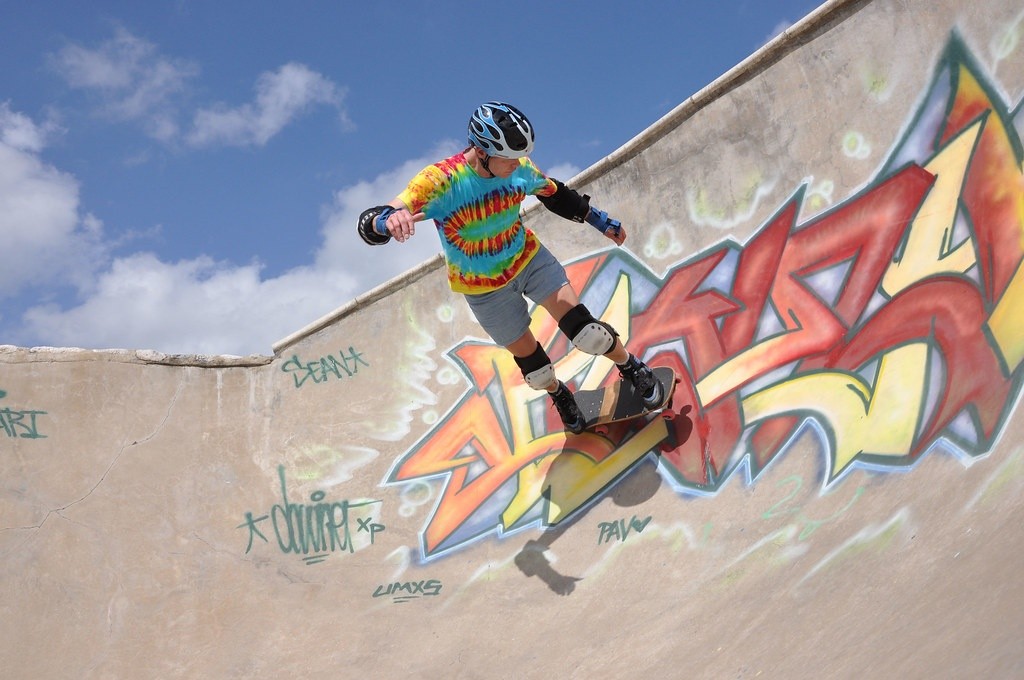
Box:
[571,364,682,435]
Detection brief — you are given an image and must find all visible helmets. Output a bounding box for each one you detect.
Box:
[467,101,534,159]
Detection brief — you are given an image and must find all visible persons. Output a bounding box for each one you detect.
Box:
[357,101,663,435]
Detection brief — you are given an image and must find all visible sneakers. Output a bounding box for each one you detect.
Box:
[546,379,586,436]
[616,354,663,410]
